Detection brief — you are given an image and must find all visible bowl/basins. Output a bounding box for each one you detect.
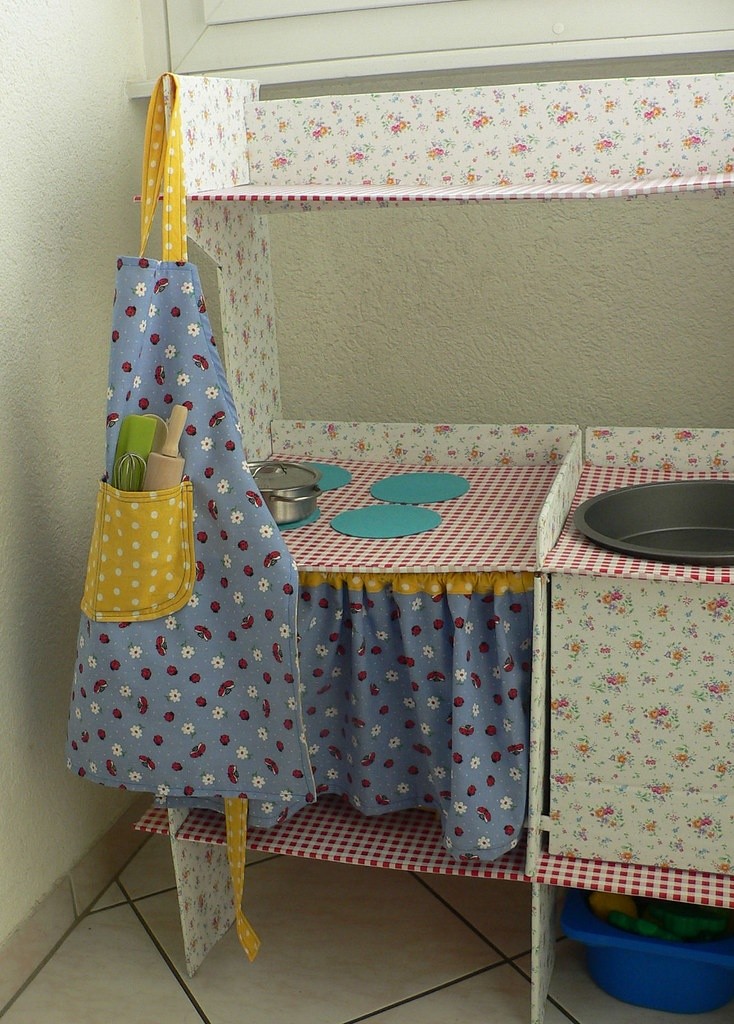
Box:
[560,889,734,1015]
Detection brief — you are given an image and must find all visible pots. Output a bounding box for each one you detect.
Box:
[246,461,323,526]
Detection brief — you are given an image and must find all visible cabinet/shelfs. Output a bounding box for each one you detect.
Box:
[133,74,734,1024]
